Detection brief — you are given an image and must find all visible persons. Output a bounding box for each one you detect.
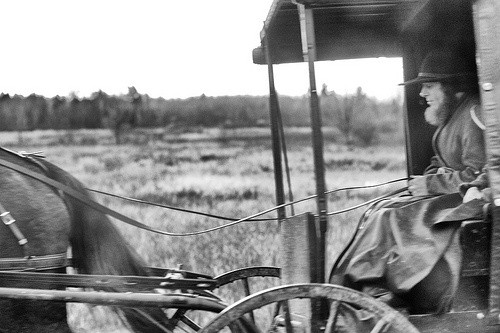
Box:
[328,47,493,313]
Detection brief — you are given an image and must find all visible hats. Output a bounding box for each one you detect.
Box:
[397,41,470,86]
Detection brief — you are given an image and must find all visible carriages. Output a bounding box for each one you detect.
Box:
[0,0,499,332]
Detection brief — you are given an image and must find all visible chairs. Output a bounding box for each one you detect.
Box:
[456,201,492,278]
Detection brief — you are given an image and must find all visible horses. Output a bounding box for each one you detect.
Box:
[0,147,179,333]
